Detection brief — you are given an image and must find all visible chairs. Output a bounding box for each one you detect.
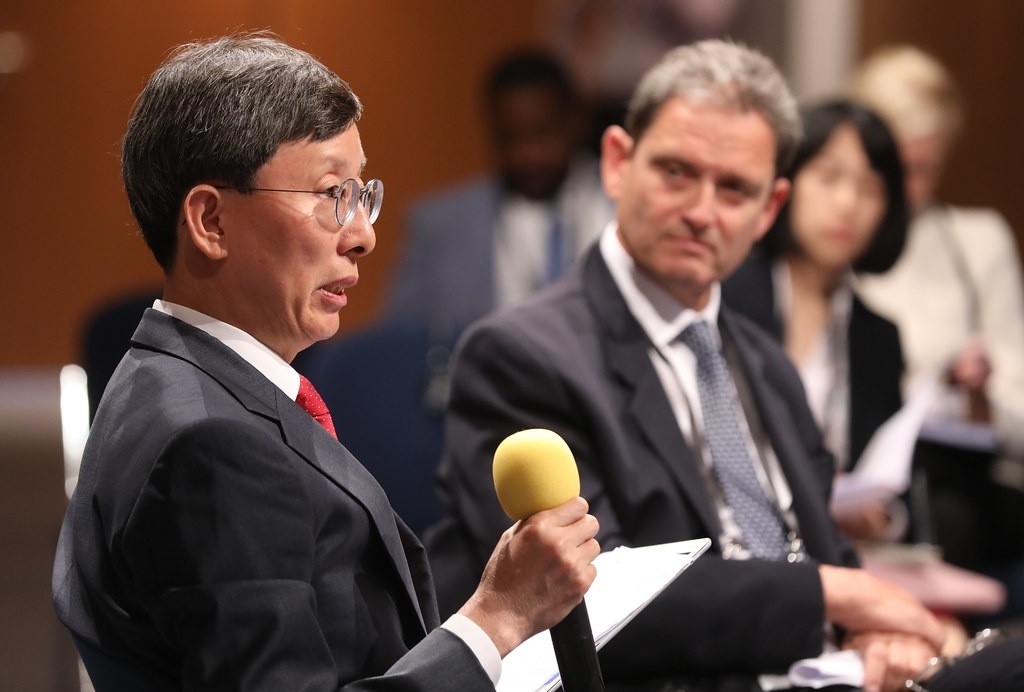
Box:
[60,320,459,692]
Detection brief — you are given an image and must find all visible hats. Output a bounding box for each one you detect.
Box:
[854,46,945,138]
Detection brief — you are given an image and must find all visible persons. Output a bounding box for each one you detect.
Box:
[421,42,1024,692]
[405,45,612,403]
[52,30,600,692]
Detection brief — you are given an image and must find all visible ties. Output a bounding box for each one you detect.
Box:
[678,319,789,564]
[295,373,338,444]
[932,209,993,429]
[543,201,569,283]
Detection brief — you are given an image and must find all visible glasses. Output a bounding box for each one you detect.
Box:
[182,178,385,228]
[905,628,1004,692]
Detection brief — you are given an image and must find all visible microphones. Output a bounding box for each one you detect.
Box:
[491,428,607,692]
